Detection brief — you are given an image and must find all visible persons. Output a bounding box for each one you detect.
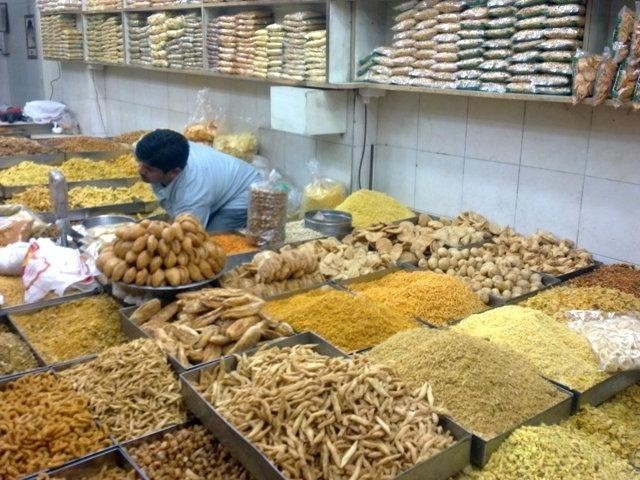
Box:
[134,129,262,233]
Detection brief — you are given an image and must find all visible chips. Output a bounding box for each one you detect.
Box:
[352,211,503,266]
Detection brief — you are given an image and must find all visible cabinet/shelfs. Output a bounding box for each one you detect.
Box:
[37,1,352,92]
[347,0,640,108]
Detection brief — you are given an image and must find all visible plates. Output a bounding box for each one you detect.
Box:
[116,266,223,292]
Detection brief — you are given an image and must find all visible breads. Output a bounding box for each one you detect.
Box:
[96,213,324,368]
